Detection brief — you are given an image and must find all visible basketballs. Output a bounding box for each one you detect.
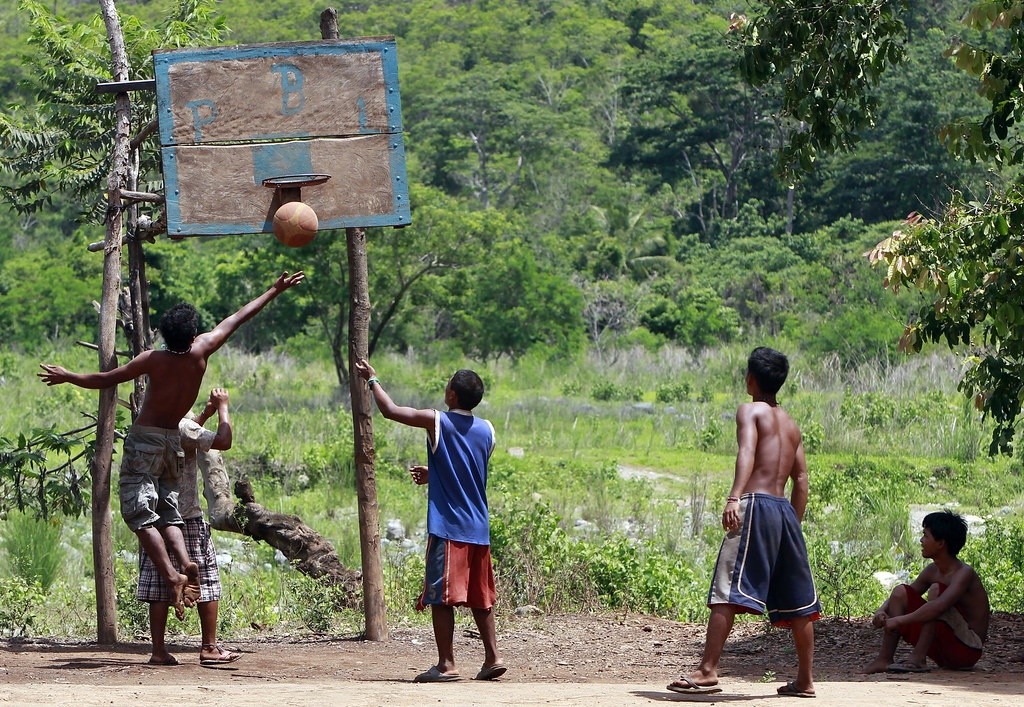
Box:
[272,201,318,246]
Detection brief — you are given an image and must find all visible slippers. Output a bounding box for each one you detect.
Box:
[666,677,722,693]
[886,659,929,672]
[414,665,461,681]
[777,681,817,697]
[953,668,973,672]
[475,663,507,680]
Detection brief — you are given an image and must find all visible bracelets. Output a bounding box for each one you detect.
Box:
[727,497,739,502]
[369,378,380,390]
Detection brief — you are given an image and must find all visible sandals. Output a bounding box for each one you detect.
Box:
[200,644,245,664]
[149,653,178,665]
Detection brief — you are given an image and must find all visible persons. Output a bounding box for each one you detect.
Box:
[668,346,820,698]
[138,388,245,665]
[36,271,305,621]
[854,509,990,674]
[355,359,507,682]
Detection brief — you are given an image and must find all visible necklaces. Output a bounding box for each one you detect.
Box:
[161,344,190,354]
